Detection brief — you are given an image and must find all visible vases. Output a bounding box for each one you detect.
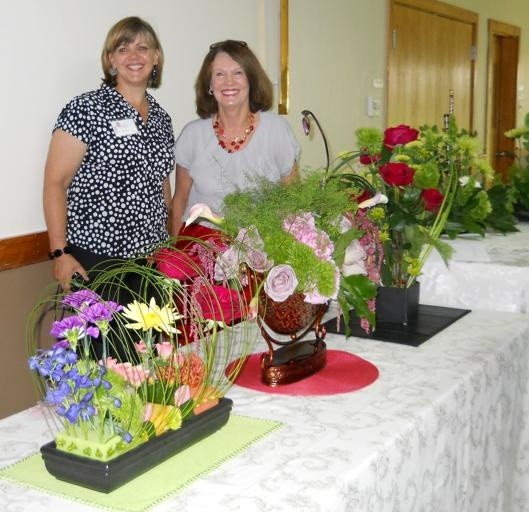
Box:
[37,396,237,496]
[373,279,421,330]
[244,265,333,388]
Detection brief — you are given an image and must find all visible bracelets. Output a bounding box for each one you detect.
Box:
[47,246,71,261]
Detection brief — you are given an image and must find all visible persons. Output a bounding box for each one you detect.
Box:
[166,38,302,348]
[41,11,177,371]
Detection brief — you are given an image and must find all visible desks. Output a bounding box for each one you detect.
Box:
[416,214,529,313]
[0,297,529,510]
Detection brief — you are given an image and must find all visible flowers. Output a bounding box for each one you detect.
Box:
[332,121,474,289]
[27,227,269,461]
[180,169,390,338]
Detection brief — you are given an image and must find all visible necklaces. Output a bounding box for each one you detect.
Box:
[210,109,255,154]
